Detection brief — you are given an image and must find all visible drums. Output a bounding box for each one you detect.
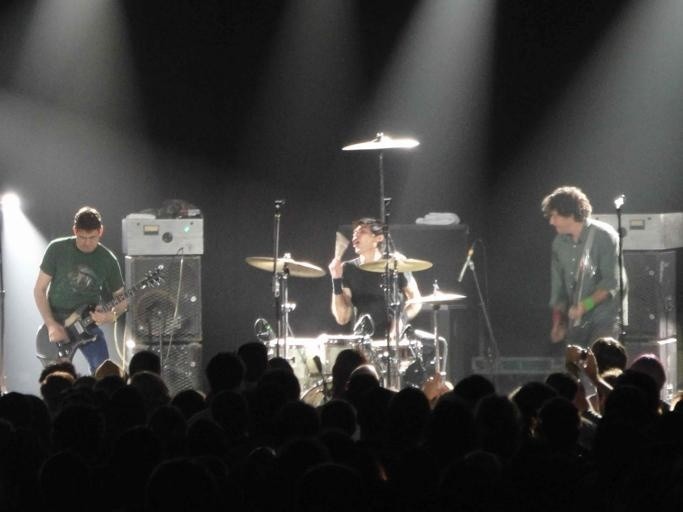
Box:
[264,335,314,386]
[369,338,422,363]
[398,356,427,390]
[299,377,333,407]
[318,333,359,372]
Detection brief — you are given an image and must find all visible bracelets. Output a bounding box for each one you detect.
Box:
[583,296,593,309]
[332,277,343,295]
[551,309,561,321]
[110,307,118,320]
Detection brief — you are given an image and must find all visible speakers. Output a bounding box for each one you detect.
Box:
[622,248,683,340]
[125,342,205,398]
[125,256,203,344]
[623,339,683,402]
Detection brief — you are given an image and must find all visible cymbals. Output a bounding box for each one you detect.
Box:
[358,251,428,276]
[411,293,465,308]
[342,132,418,150]
[244,254,326,278]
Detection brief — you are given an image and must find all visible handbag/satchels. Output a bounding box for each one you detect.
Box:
[157,199,202,218]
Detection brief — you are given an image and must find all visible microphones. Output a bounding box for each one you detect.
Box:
[457,239,478,284]
[615,193,626,209]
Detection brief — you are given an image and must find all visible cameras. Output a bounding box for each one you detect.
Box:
[578,349,588,362]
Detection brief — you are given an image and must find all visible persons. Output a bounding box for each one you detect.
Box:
[541,186,628,396]
[34,205,129,376]
[1,334,683,511]
[327,217,423,340]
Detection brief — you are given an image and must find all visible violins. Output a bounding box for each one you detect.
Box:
[35,264,163,365]
[550,247,588,341]
